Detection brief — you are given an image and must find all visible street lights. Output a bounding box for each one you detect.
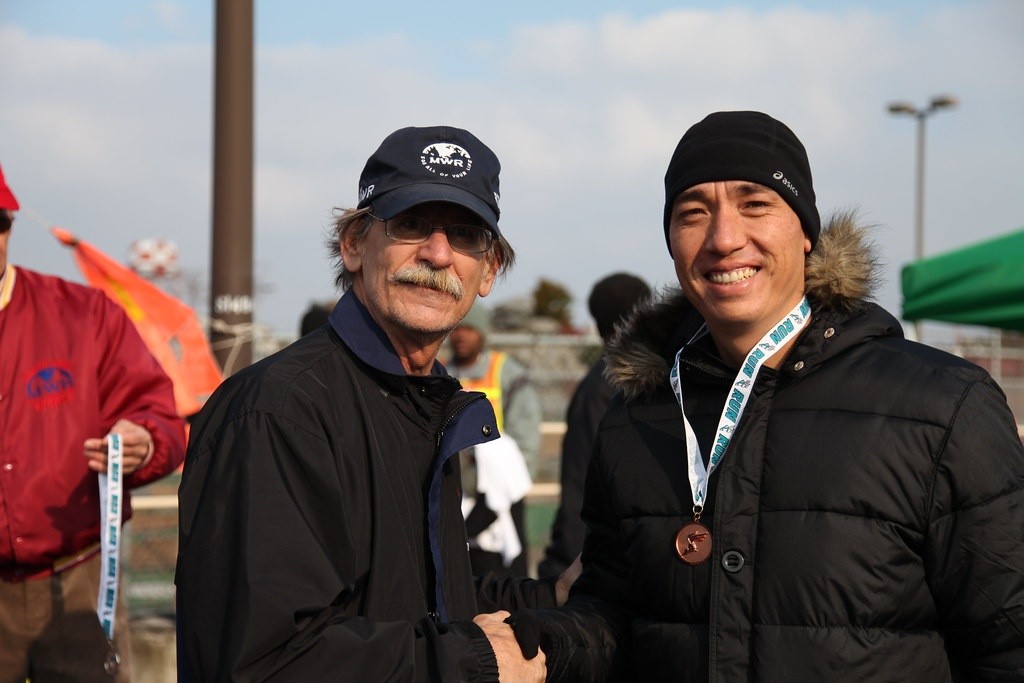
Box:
[886,93,960,344]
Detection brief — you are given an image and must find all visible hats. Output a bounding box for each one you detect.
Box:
[455,305,487,348]
[357,126,501,241]
[0,166,19,210]
[590,274,651,321]
[664,110,820,258]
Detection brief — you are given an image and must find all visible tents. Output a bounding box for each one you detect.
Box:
[901,228,1024,342]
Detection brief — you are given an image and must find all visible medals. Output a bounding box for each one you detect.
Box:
[676,522,712,563]
[103,652,120,675]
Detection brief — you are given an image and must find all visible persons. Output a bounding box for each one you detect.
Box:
[0,164,188,683]
[175,112,1024,683]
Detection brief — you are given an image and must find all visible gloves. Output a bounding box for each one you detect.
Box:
[504,608,554,663]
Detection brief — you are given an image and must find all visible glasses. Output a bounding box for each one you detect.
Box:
[0,213,15,233]
[367,212,496,255]
